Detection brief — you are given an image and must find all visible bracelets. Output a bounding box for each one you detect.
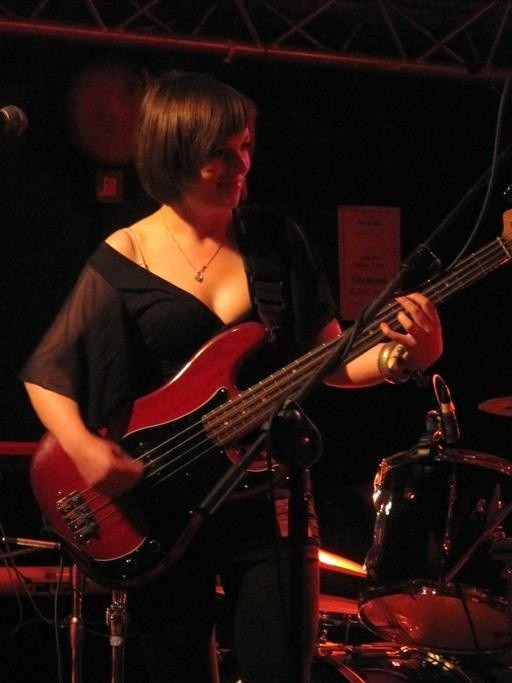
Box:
[391,343,428,381]
[378,340,411,385]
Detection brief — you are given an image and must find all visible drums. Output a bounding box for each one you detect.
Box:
[312,641,472,682]
[316,596,379,644]
[357,447,511,655]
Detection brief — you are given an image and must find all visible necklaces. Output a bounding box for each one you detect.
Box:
[159,206,229,282]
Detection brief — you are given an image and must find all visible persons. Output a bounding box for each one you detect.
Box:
[17,68,445,681]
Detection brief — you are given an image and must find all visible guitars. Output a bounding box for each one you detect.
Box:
[30,210,512,575]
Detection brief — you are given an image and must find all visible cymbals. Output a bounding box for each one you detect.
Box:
[479,397,512,417]
